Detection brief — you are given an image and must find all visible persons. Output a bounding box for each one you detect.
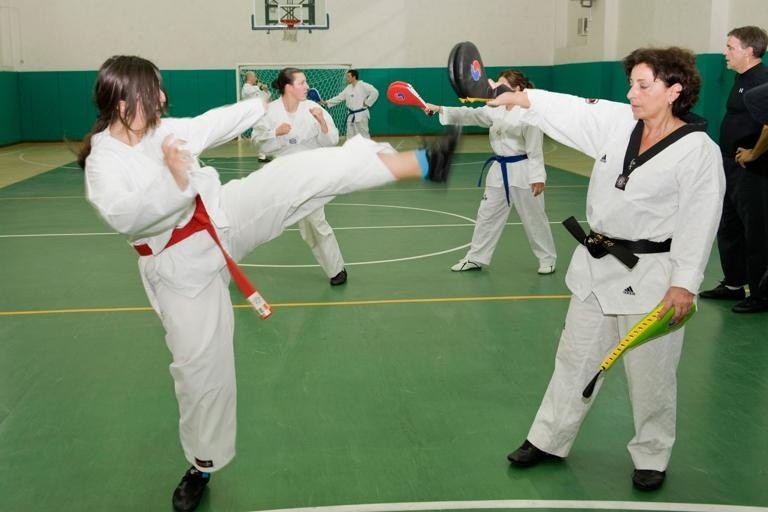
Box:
[241,71,271,163]
[250,67,347,287]
[424,69,558,275]
[484,44,728,493]
[698,25,768,315]
[74,54,466,512]
[319,69,380,141]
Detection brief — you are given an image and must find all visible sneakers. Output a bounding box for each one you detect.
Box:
[173,464,210,512]
[537,265,555,274]
[425,117,461,183]
[732,296,768,313]
[330,268,347,284]
[507,439,561,463]
[451,260,482,271]
[631,469,666,492]
[698,284,746,301]
